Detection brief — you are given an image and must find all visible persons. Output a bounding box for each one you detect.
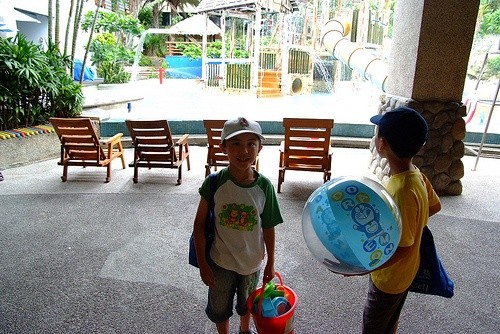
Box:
[323,105,441,334]
[192,117,283,334]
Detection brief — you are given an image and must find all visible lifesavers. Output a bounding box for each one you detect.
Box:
[463,96,477,124]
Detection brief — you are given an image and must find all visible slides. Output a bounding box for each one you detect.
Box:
[321,7,389,93]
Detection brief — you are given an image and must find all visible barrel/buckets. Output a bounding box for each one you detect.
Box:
[247,272,297,334]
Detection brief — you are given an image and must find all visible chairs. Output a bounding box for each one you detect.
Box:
[49,116,126,184]
[203,119,259,179]
[125,119,190,186]
[277,117,334,195]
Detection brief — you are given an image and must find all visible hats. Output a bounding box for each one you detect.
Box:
[220,116,266,142]
[370,104,427,144]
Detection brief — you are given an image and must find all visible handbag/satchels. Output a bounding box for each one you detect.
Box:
[407,224,454,298]
[188,173,216,267]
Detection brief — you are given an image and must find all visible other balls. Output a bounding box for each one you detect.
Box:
[300,175,402,275]
